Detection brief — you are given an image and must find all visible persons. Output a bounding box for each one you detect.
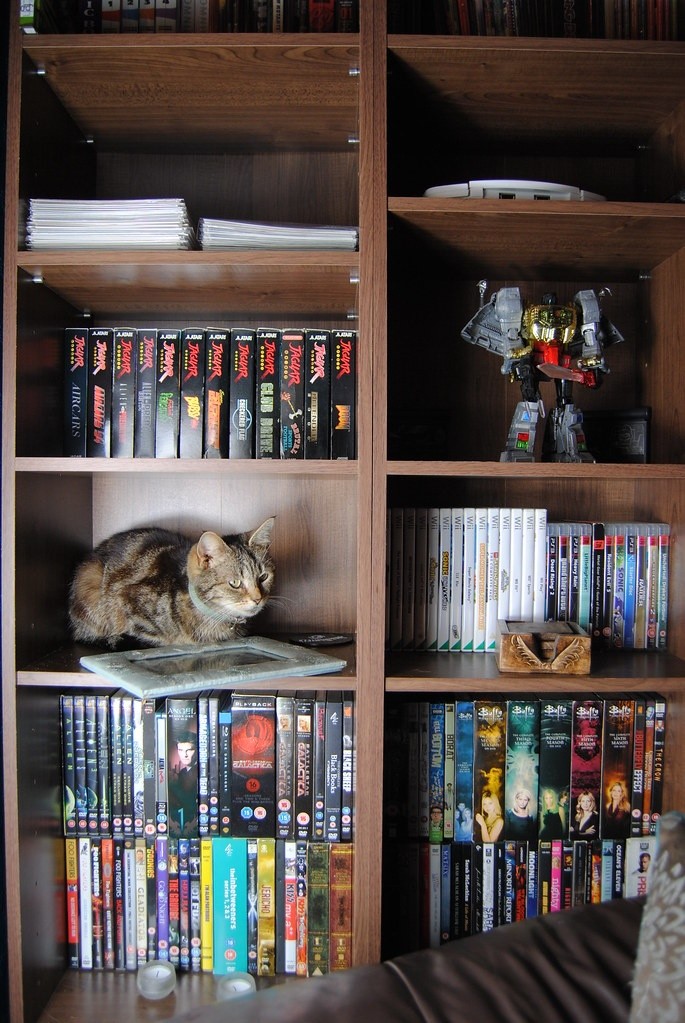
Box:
[505,789,533,839]
[570,791,598,835]
[430,806,442,831]
[539,789,565,841]
[299,718,309,731]
[558,791,567,807]
[633,853,651,874]
[180,844,188,859]
[461,808,472,834]
[136,849,146,864]
[169,856,177,873]
[457,800,465,823]
[476,790,503,842]
[168,730,197,789]
[279,714,291,731]
[455,809,460,832]
[606,781,630,819]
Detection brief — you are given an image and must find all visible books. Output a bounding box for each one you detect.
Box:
[387,508,668,652]
[63,327,355,460]
[19,0,685,37]
[386,692,667,951]
[59,689,355,978]
[25,198,359,252]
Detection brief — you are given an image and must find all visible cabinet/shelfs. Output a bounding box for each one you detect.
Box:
[0,0,685,1023]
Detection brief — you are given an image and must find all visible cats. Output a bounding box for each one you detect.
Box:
[67,514,275,645]
